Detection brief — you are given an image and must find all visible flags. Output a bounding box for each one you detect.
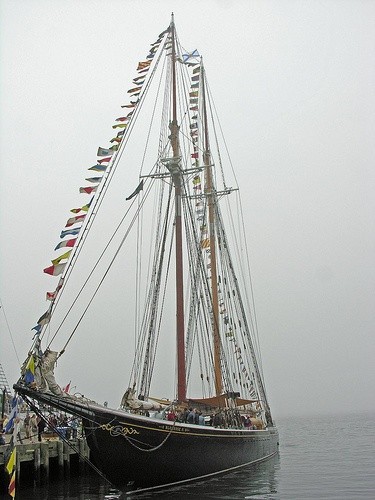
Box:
[3,394,17,500]
[26,20,267,420]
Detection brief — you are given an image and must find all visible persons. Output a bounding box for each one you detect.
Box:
[24,412,78,440]
[169,406,253,431]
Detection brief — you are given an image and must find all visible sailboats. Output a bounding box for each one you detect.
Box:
[12,26,282,494]
